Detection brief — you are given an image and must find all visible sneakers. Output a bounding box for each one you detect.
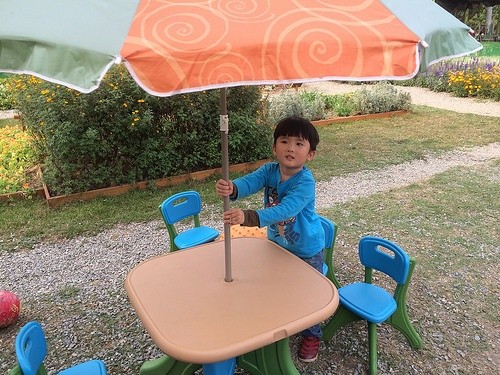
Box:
[298,336,320,362]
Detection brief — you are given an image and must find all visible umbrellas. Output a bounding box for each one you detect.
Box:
[0,0,483,283]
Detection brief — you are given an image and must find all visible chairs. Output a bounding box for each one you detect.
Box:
[7,321,107,375]
[158,190,220,253]
[315,214,341,289]
[314,238,422,373]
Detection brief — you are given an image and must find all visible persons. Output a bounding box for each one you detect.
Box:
[216,116,326,363]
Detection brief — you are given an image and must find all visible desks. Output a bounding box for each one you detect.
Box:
[124,238,340,375]
